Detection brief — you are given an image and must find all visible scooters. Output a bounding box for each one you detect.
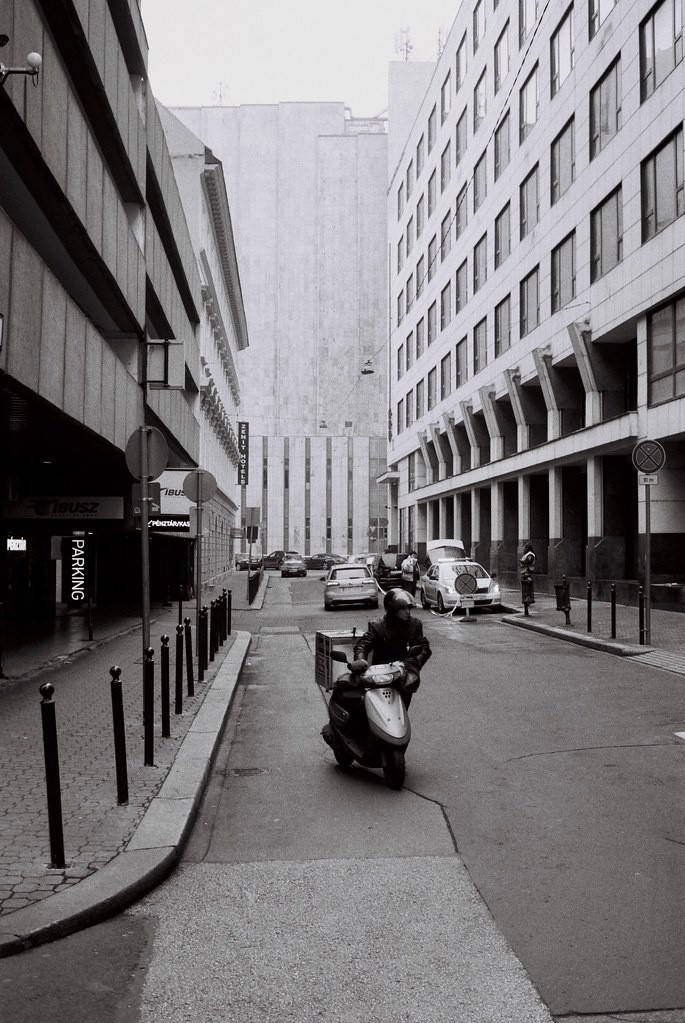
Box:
[320,644,425,790]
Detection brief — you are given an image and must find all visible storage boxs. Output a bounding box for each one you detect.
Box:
[315,629,367,688]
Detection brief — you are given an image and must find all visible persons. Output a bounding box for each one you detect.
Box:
[353,588,433,712]
[519,544,535,580]
[401,551,420,598]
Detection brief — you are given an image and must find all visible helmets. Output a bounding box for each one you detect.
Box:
[384,588,416,612]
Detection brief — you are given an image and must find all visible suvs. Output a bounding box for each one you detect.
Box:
[371,553,409,591]
[259,550,298,570]
[280,554,308,577]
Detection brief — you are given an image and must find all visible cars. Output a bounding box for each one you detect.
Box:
[319,564,378,612]
[346,551,378,571]
[235,554,262,571]
[305,553,347,570]
[419,539,502,615]
